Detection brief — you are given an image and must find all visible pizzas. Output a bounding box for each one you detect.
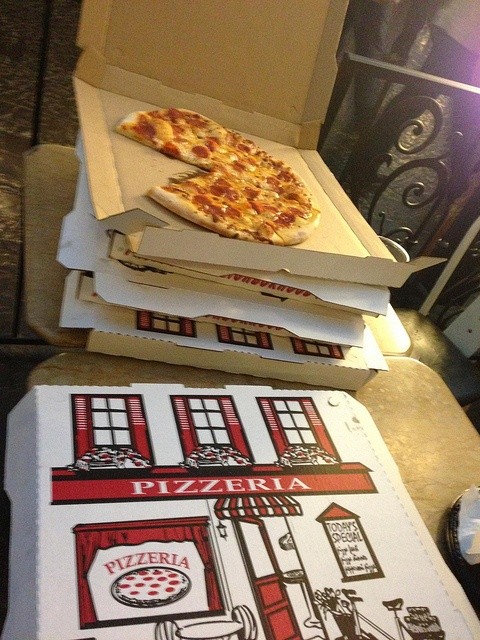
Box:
[114,106,324,246]
